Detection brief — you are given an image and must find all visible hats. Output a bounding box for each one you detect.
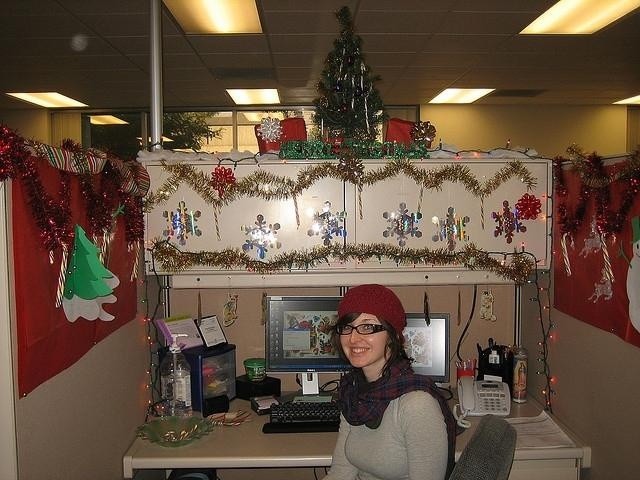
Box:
[338,284,406,335]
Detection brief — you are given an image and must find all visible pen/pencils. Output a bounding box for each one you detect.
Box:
[477,338,513,368]
[456,358,477,371]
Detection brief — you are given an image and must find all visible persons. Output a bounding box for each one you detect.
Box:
[319,282,455,480]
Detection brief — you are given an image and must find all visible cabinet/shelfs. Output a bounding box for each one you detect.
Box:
[158,344,236,412]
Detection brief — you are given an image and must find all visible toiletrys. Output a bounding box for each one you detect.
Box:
[161,332,192,417]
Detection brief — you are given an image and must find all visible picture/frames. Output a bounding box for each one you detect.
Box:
[194,315,228,348]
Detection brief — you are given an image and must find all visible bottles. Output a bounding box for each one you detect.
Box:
[512,345,529,404]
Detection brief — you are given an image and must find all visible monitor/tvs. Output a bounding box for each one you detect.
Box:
[401,313,450,383]
[265,296,356,402]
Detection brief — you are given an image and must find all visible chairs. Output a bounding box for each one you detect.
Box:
[447,414,516,480]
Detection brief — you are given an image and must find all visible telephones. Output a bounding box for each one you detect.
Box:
[457,375,511,416]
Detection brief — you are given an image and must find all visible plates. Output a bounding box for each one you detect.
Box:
[135,415,213,449]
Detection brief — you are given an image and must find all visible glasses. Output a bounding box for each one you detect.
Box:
[335,321,389,335]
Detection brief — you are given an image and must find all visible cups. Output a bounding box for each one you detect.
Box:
[242,357,268,382]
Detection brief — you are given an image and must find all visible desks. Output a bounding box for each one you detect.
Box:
[123,391,592,480]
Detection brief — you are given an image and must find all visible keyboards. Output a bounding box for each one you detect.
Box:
[263,402,340,434]
